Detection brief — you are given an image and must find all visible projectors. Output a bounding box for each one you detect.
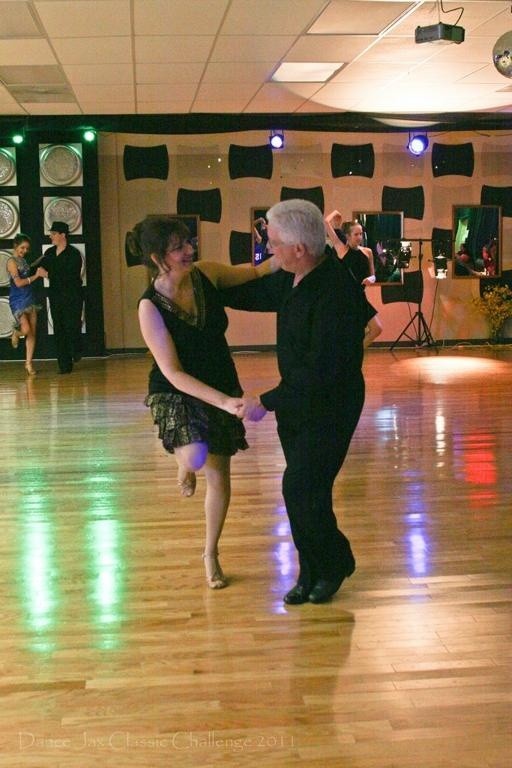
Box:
[416,22,466,47]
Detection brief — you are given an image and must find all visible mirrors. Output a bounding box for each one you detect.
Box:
[452,205,502,280]
[149,213,201,282]
[351,210,404,285]
[251,208,274,266]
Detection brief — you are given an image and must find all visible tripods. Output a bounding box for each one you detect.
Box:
[389,241,437,349]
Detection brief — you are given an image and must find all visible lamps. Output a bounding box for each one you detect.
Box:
[407,132,431,156]
[268,127,286,151]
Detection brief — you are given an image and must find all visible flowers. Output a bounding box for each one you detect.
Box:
[472,284,511,343]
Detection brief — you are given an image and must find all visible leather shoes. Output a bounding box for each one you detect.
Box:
[284,558,355,604]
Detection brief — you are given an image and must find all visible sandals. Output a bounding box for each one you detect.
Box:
[178,472,196,497]
[202,552,226,589]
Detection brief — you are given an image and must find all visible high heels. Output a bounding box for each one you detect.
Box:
[25,363,37,376]
[9,330,18,349]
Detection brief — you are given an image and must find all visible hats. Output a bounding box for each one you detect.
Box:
[48,222,70,233]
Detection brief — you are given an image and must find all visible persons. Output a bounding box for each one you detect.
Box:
[241,196,373,607]
[456,242,473,266]
[250,214,268,266]
[38,218,83,374]
[124,212,285,592]
[324,206,381,352]
[6,231,41,376]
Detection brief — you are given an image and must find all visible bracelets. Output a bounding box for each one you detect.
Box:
[26,276,33,286]
